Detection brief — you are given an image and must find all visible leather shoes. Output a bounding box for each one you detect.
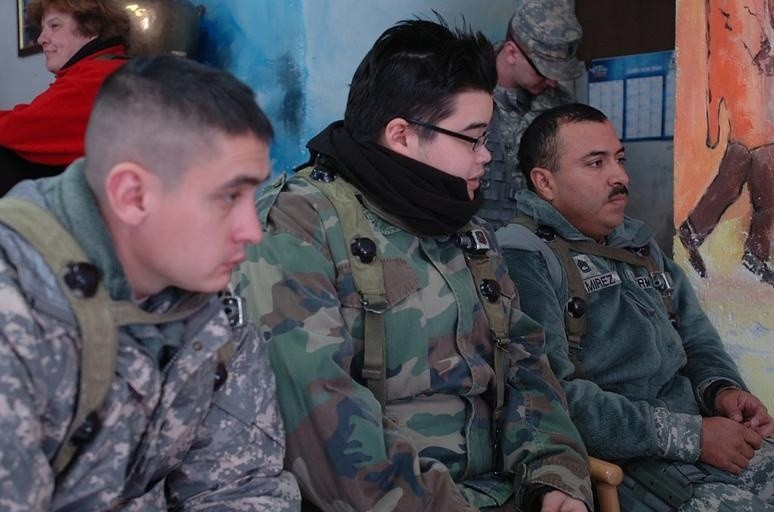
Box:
[740,250,774,287]
[677,219,707,278]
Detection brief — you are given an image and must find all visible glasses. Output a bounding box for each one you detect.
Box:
[405,115,489,152]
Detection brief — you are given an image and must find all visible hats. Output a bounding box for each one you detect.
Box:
[511,0,585,81]
